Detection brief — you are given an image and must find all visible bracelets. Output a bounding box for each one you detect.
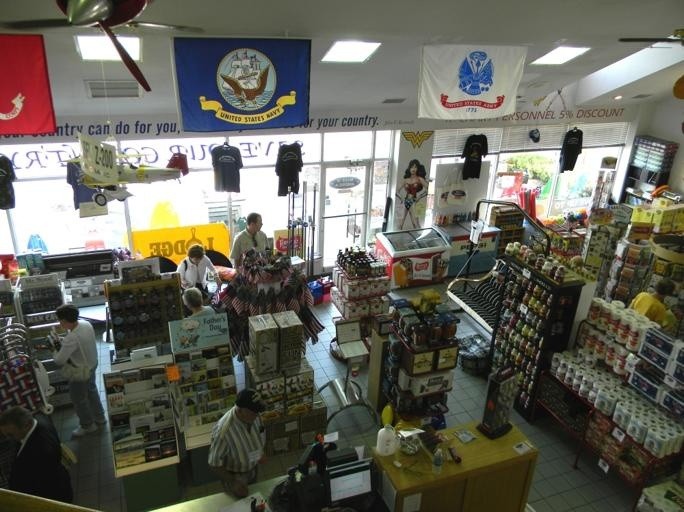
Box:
[226,477,237,485]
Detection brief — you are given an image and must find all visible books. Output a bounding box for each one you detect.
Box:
[220,491,272,512]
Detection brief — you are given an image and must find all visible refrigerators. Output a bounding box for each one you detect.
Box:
[433,224,501,279]
[374,227,449,289]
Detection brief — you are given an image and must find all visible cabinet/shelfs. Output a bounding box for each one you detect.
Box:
[0,130,684,512]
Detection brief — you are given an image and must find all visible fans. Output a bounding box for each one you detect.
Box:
[603,25,684,100]
[0,1,208,97]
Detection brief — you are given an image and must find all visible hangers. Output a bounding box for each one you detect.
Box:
[222,141,229,147]
[571,126,577,129]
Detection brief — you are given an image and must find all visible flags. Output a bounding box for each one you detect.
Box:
[0,33,56,137]
[417,43,529,119]
[172,31,312,132]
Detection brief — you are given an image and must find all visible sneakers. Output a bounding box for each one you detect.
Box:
[93,418,108,426]
[71,423,97,436]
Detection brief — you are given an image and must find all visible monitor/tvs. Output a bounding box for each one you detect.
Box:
[327,457,378,508]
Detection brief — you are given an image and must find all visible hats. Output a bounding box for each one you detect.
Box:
[235,388,268,413]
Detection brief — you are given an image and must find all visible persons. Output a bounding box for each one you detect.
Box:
[626,278,675,327]
[182,287,218,359]
[661,303,683,339]
[207,388,267,499]
[395,159,428,230]
[230,213,270,272]
[48,303,108,438]
[176,244,222,307]
[0,406,75,506]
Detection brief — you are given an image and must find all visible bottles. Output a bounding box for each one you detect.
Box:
[20,285,59,314]
[392,305,458,345]
[435,209,475,226]
[336,245,387,276]
[110,282,176,345]
[432,448,443,476]
[489,266,552,411]
[504,241,566,284]
